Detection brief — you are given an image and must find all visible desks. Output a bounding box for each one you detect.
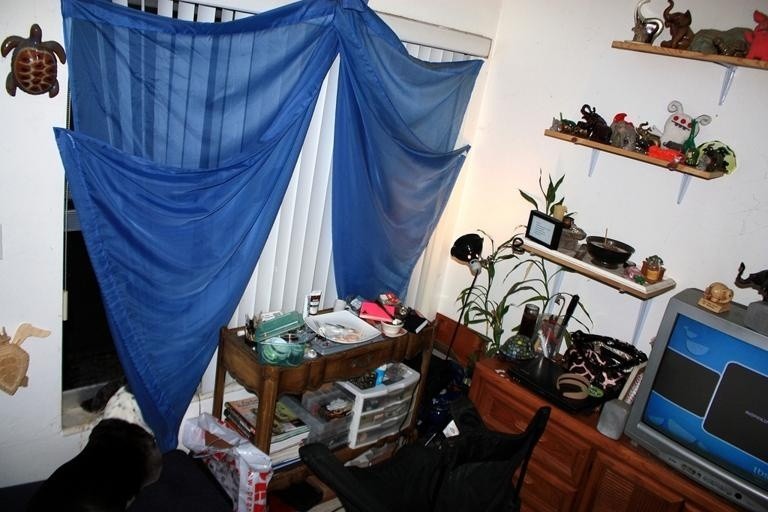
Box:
[211,300,440,491]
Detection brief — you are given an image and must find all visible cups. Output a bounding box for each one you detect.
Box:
[333,299,351,312]
[381,320,404,335]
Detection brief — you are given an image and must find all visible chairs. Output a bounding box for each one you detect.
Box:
[428,311,490,372]
[297,390,552,511]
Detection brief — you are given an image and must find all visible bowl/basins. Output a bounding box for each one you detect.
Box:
[262,337,290,364]
[586,236,635,269]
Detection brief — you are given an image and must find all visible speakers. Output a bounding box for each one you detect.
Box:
[597,400,631,442]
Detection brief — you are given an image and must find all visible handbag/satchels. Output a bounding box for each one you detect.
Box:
[403,399,514,510]
[562,330,648,395]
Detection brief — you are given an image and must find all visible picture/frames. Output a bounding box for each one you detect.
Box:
[524,209,562,250]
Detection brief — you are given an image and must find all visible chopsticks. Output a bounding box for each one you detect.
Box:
[313,319,327,342]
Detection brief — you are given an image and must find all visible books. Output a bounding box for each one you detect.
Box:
[224,395,311,471]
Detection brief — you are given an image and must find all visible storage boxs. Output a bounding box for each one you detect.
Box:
[255,312,308,368]
[284,360,421,449]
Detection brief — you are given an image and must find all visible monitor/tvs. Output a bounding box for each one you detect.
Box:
[624,288,768,512]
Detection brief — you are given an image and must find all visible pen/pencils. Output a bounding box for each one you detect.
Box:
[376,300,395,320]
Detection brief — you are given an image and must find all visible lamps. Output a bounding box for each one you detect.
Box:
[443,234,484,364]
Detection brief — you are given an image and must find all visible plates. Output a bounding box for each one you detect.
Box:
[377,324,408,338]
[303,310,382,344]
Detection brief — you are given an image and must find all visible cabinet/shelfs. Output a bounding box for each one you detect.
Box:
[466,355,737,512]
[515,40,767,300]
